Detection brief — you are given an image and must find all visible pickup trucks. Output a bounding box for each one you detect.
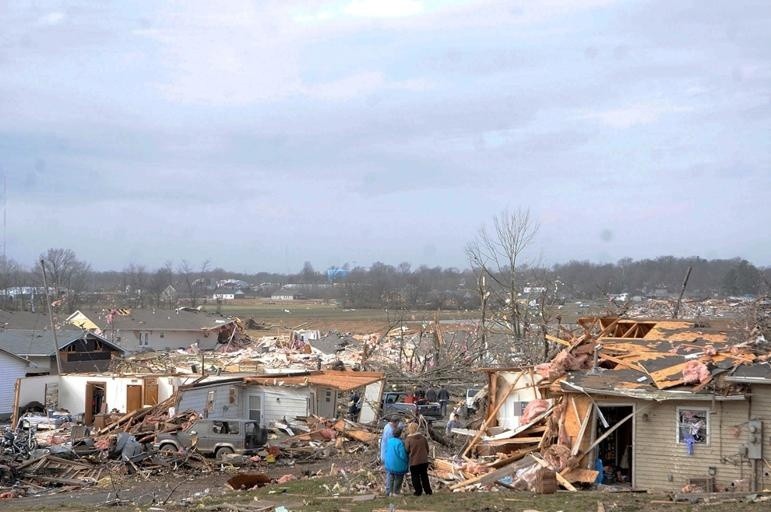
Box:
[357,391,442,418]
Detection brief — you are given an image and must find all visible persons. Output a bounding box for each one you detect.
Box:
[379,415,400,496]
[347,384,450,423]
[385,428,409,498]
[446,414,465,448]
[405,422,434,496]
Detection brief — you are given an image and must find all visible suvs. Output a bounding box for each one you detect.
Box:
[153,419,265,458]
